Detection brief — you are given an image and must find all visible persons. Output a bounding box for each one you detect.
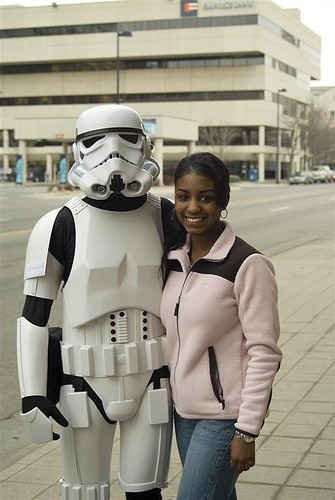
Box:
[159,153,282,500]
[16,103,187,500]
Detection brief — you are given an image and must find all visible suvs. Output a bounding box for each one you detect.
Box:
[312,165,332,184]
[11,164,46,183]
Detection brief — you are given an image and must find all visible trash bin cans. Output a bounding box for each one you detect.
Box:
[248,169,258,181]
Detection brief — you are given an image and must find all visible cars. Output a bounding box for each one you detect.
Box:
[287,171,313,185]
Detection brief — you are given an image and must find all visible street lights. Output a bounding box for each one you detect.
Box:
[275,88,287,183]
[115,30,132,104]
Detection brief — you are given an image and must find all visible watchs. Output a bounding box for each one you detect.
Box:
[236,431,254,442]
